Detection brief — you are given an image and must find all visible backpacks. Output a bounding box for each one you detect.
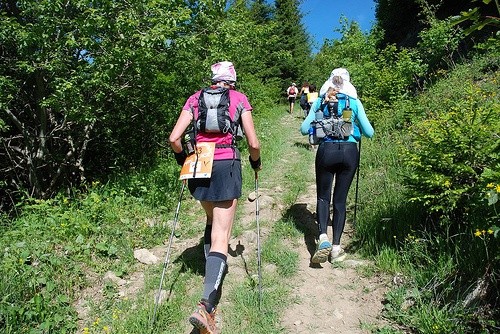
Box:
[315,94,353,140]
[197,87,235,134]
[307,92,318,103]
[289,86,296,95]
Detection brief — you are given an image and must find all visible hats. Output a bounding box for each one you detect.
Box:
[211,61,236,86]
[319,68,358,99]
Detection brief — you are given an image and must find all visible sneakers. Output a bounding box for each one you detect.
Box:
[331,249,347,264]
[189,303,217,334]
[311,236,332,263]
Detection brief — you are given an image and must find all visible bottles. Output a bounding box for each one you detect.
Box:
[316,109,326,137]
[309,122,318,144]
[343,106,352,136]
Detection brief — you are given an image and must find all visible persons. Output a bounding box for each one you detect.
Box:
[299,67,374,264]
[168,62,262,334]
[287,83,298,114]
[300,81,319,117]
